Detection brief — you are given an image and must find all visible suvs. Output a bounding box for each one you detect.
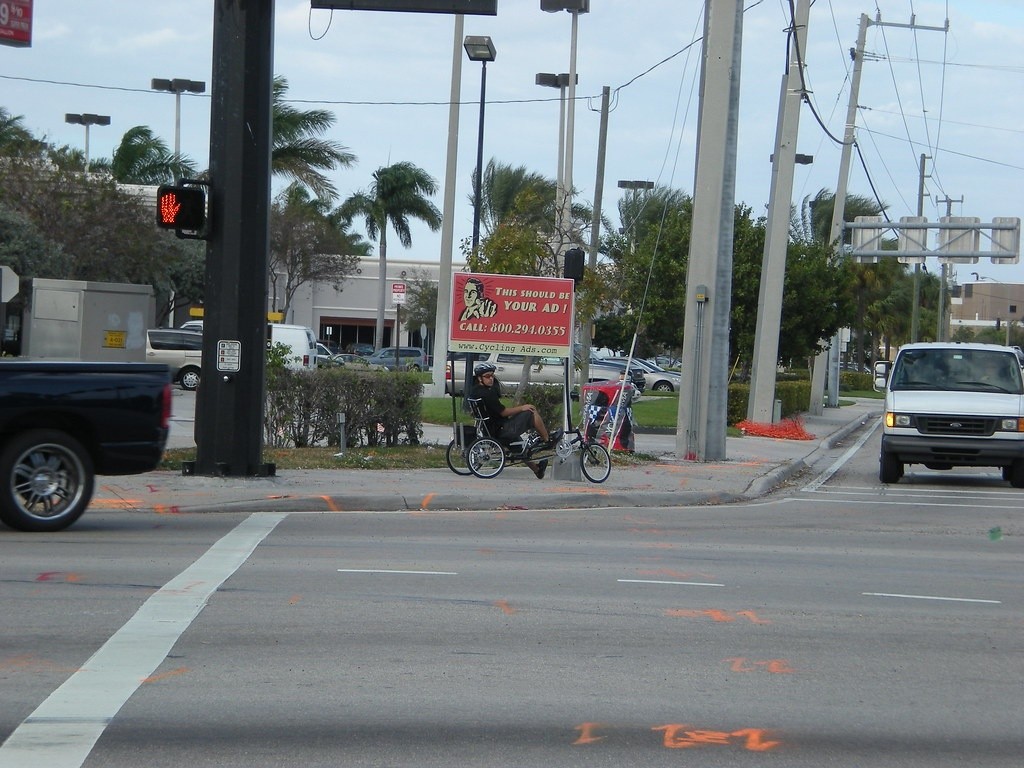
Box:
[364,346,429,372]
[147,329,204,391]
[445,346,646,397]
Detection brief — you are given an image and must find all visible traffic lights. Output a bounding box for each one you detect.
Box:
[155,185,204,229]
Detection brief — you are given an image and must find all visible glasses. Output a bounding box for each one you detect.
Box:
[482,373,495,379]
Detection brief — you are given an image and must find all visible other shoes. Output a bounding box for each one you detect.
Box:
[542,427,563,450]
[534,459,548,479]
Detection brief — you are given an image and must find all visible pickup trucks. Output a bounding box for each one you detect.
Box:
[0,362,173,532]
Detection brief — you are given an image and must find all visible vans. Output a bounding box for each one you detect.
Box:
[874,339,1024,488]
[180,320,318,391]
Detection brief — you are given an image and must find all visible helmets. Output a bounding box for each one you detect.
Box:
[619,369,633,383]
[474,364,496,376]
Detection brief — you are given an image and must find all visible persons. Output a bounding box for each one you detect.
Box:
[469,362,563,479]
[981,357,1008,381]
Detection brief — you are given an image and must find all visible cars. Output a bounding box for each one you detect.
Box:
[601,356,682,392]
[839,362,871,373]
[317,340,390,372]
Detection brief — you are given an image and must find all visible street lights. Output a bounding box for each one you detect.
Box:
[618,180,654,255]
[980,276,1010,346]
[151,77,206,185]
[64,112,110,173]
[540,0,590,280]
[535,72,578,265]
[463,36,497,404]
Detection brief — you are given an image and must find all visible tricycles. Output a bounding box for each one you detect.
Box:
[446,399,612,487]
